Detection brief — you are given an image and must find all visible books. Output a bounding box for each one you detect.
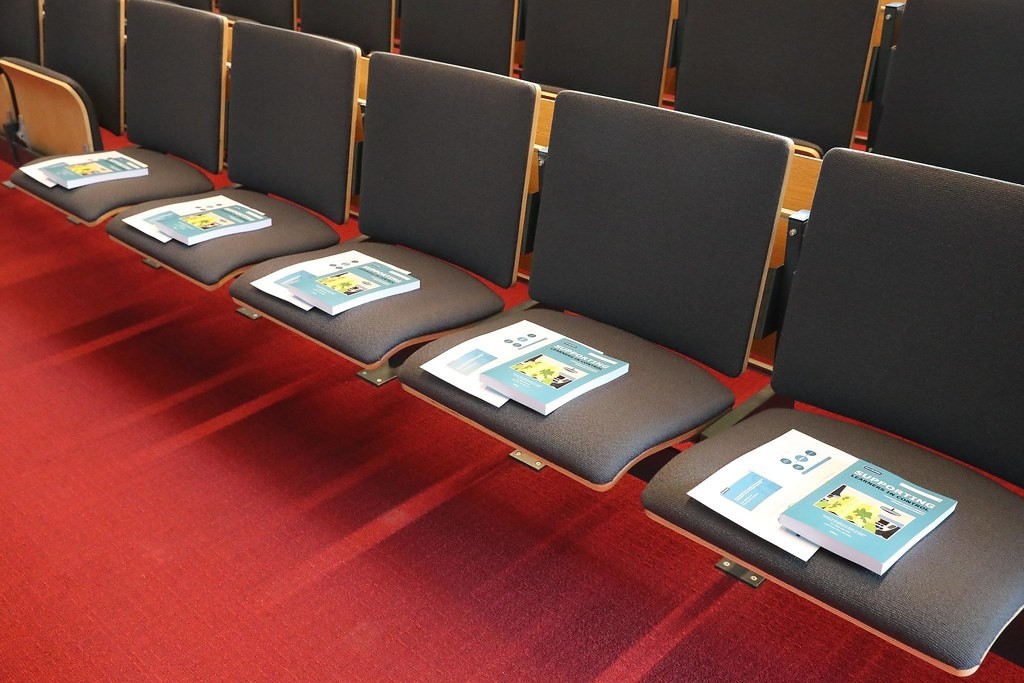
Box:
[39,153,149,189]
[779,457,958,576]
[156,205,272,246]
[479,338,630,416]
[288,260,421,316]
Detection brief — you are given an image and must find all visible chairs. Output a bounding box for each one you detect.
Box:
[0,0,1024,676]
